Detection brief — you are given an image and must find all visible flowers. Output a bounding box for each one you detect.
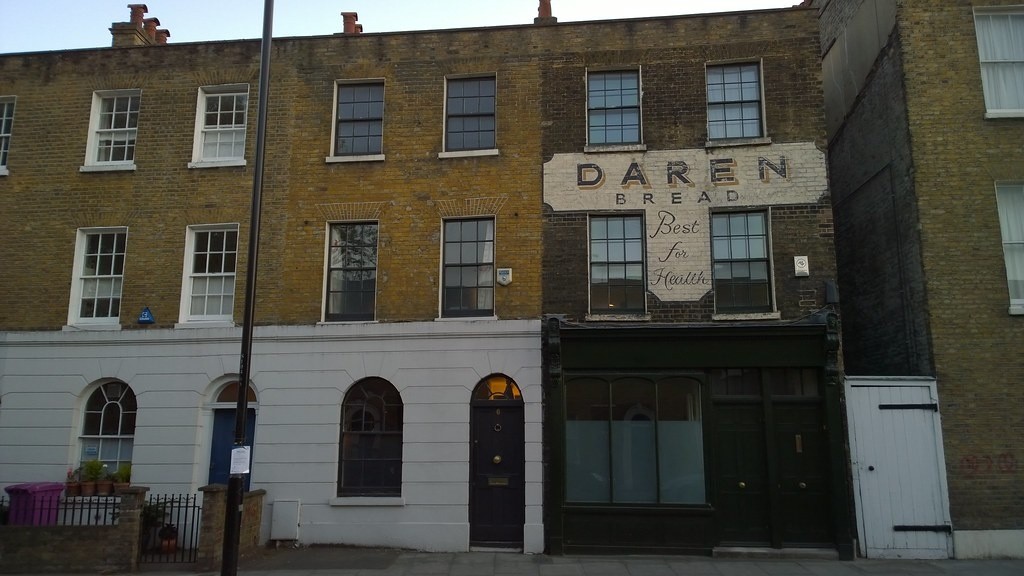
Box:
[158,527,177,538]
[64,467,80,483]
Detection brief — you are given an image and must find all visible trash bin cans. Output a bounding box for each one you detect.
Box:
[5,482,65,526]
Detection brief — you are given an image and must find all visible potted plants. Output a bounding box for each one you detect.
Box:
[80,459,98,495]
[112,463,130,495]
[97,468,112,497]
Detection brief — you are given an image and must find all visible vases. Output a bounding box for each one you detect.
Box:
[65,483,80,496]
[162,538,176,552]
